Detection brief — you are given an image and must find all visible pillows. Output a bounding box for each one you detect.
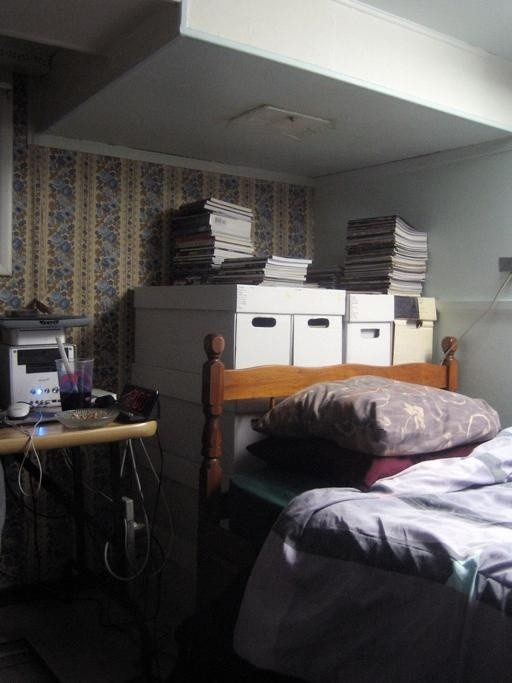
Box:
[246,374,501,492]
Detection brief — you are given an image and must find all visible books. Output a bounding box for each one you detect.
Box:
[167,196,428,298]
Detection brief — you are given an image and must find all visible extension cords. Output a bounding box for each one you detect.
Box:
[115,494,134,572]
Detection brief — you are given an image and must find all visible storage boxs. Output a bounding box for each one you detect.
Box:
[127,281,437,640]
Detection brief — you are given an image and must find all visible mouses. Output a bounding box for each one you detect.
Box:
[7,402,30,419]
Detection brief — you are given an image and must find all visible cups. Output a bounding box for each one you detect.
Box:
[55,357,95,408]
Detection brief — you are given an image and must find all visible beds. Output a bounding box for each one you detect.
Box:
[197,332,512,682]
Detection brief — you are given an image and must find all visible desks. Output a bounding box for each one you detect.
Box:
[0,409,157,682]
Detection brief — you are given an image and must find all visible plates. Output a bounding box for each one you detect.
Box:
[54,408,118,429]
